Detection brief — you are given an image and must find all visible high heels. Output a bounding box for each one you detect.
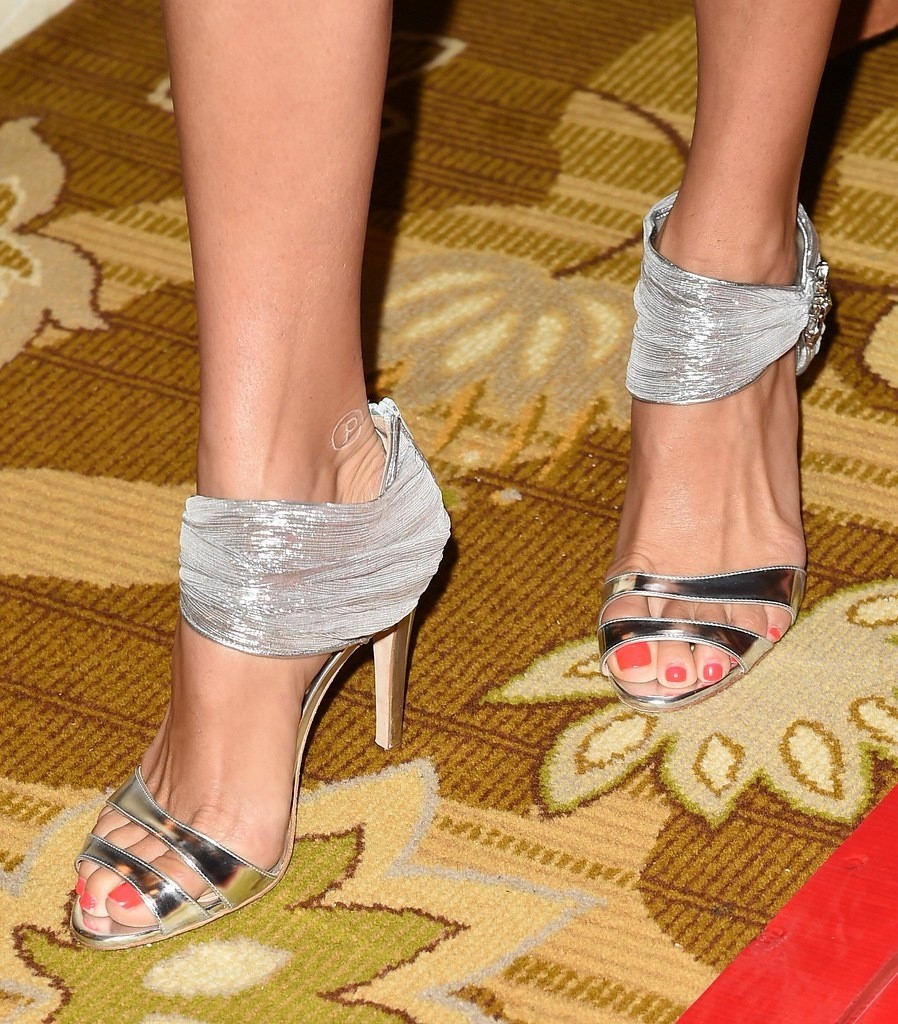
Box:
[67,398,450,951]
[594,186,832,715]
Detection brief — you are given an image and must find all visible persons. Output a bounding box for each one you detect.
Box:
[68,1,846,948]
